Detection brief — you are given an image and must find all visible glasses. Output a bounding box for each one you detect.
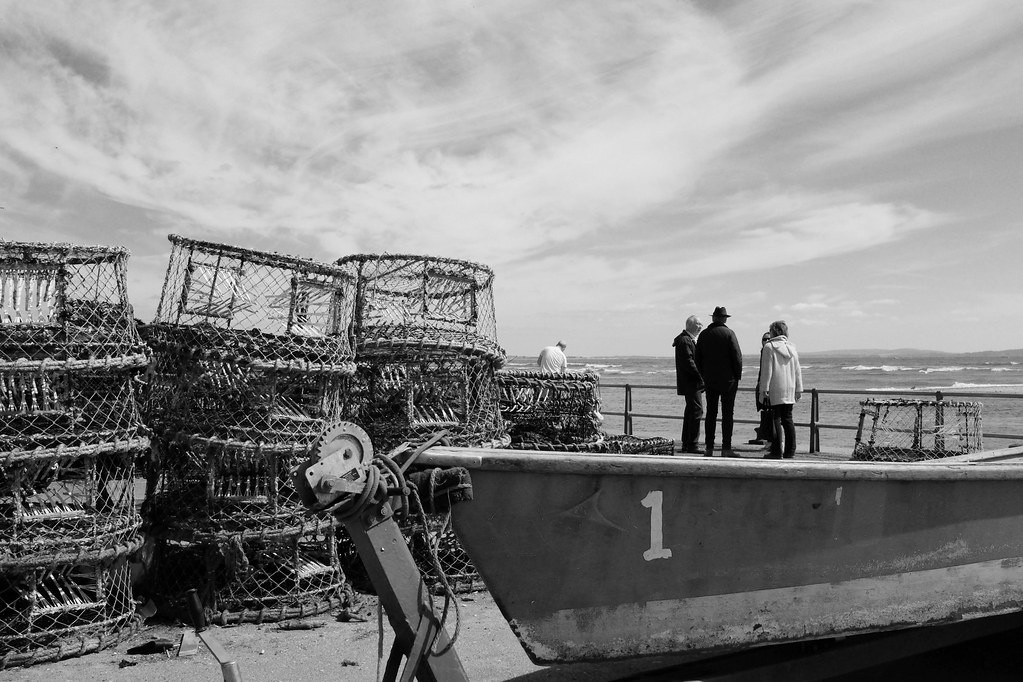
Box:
[763,338,770,341]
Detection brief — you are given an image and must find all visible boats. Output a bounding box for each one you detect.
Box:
[401,430,1023,666]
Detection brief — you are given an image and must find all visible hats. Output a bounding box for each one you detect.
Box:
[710,307,730,317]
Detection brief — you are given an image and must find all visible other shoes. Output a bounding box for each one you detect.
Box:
[704,450,712,456]
[722,450,740,457]
[763,451,783,459]
[784,453,794,458]
[682,447,705,453]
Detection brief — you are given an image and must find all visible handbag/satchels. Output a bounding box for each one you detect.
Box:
[760,398,777,440]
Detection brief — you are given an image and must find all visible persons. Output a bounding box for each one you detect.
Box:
[538,340,567,374]
[749,320,802,459]
[672,315,704,453]
[694,305,743,457]
